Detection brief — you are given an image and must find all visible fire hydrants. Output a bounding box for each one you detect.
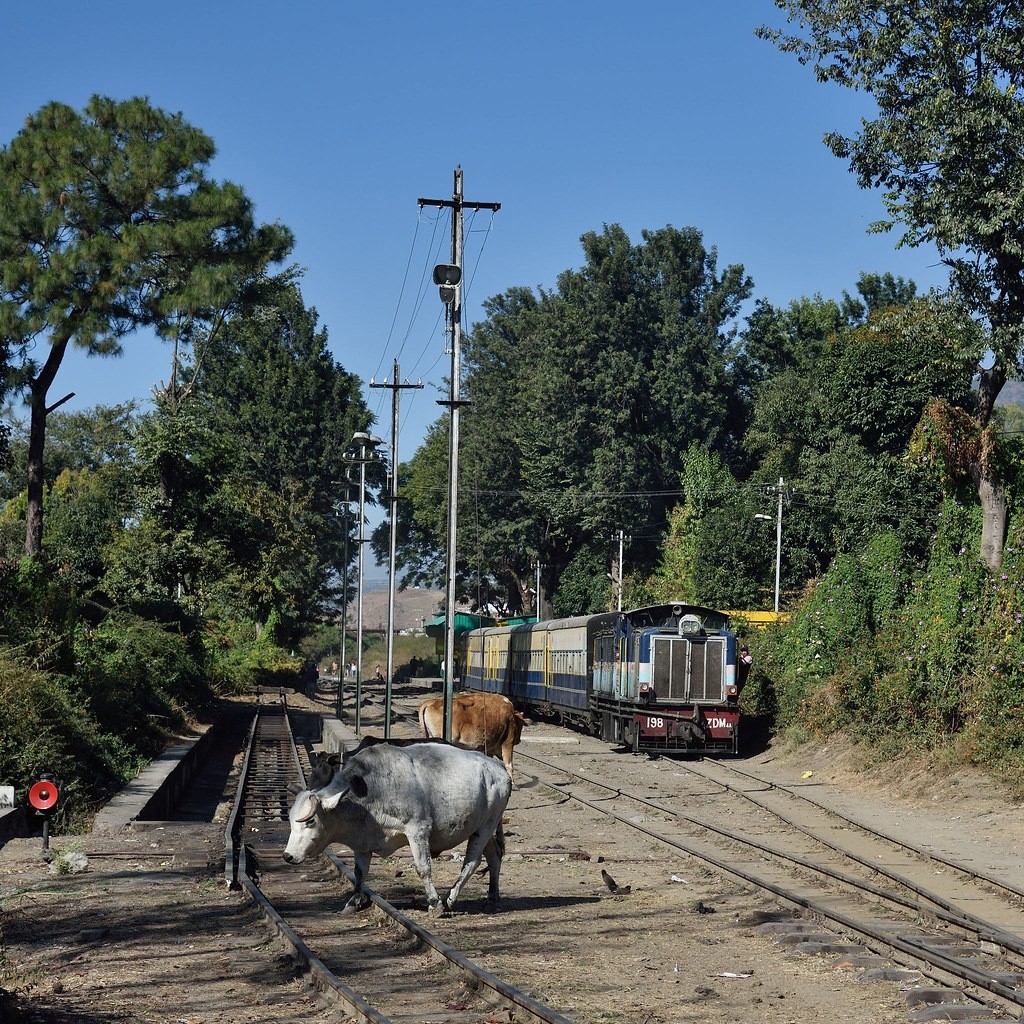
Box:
[29,774,64,848]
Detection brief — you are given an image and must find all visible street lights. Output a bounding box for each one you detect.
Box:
[352,430,384,739]
[754,513,782,615]
[332,498,358,717]
[431,262,465,740]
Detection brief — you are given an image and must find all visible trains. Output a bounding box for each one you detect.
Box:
[461,600,755,756]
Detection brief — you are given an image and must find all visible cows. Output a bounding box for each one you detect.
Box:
[279,689,528,920]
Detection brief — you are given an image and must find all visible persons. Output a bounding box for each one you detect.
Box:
[453,659,457,680]
[331,660,357,678]
[440,659,445,680]
[375,664,387,683]
[410,655,424,678]
[727,646,753,705]
